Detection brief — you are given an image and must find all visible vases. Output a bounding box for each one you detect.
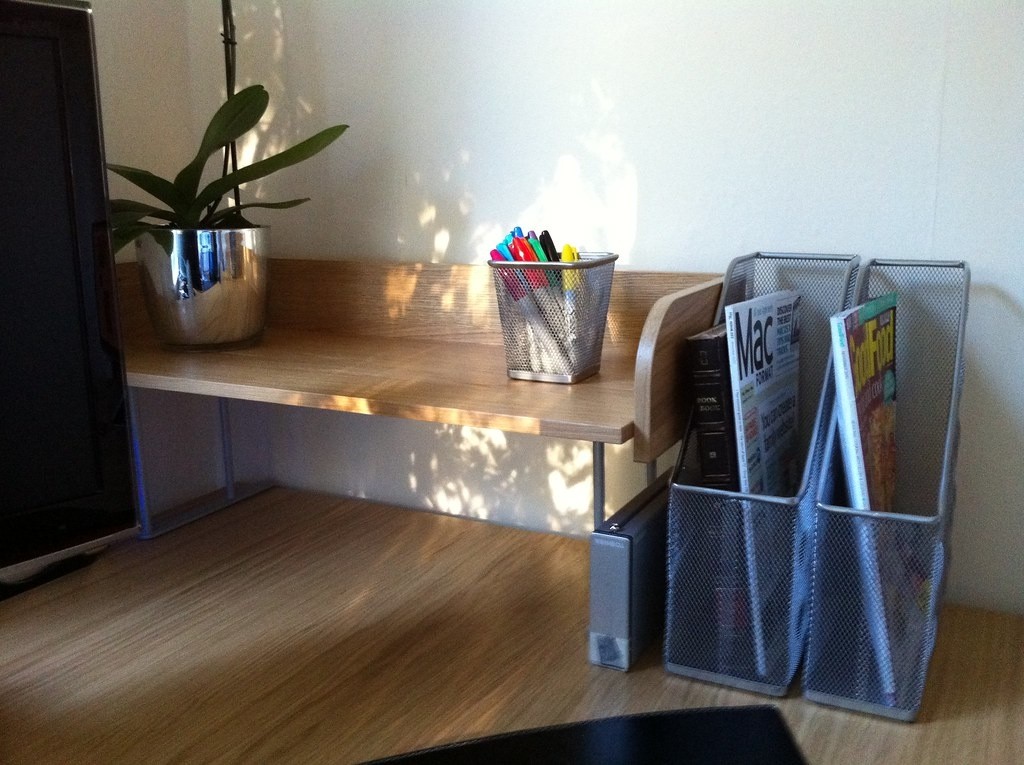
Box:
[104,83,352,353]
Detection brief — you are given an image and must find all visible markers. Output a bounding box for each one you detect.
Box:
[488,226,604,373]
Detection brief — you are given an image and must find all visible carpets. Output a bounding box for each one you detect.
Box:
[352,700,814,765]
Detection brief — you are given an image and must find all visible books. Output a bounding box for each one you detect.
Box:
[830,290,927,692]
[685,287,804,678]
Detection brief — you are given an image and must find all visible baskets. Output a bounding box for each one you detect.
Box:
[665,252,972,722]
[491,253,617,385]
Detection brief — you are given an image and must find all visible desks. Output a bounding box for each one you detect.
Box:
[114,258,748,544]
